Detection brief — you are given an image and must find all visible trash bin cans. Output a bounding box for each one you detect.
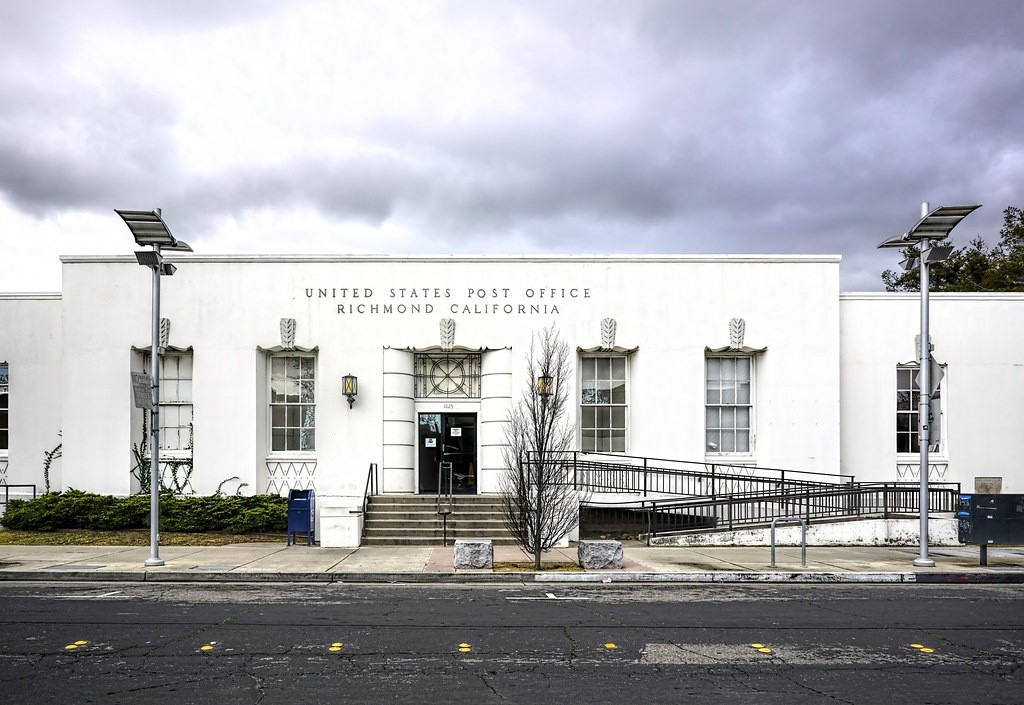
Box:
[287,488,316,547]
[958,494,1024,545]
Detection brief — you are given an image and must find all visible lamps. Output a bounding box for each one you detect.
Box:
[538,374,554,404]
[342,373,358,409]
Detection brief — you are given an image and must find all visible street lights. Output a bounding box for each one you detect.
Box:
[113,205,197,566]
[877,202,985,563]
